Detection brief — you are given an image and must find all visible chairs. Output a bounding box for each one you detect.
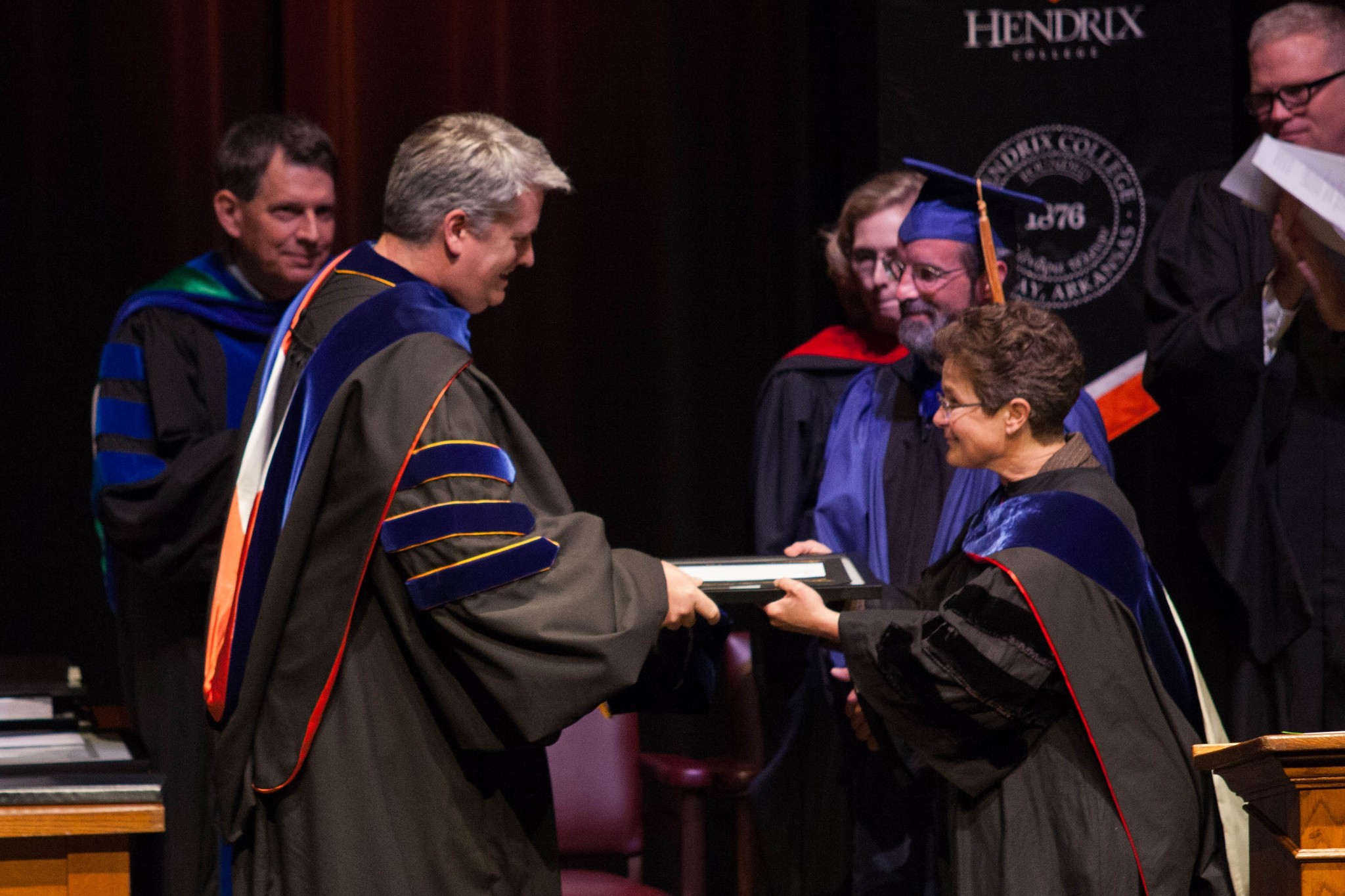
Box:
[548,703,713,895]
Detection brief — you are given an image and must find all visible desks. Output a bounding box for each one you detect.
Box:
[0,696,166,896]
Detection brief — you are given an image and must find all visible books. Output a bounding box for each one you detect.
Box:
[660,553,884,603]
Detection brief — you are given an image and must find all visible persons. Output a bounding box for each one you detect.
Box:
[747,168,1123,896]
[86,114,346,896]
[764,305,1251,896]
[1137,5,1345,736]
[183,112,722,896]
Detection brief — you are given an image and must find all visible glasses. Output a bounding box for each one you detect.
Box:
[1242,70,1344,117]
[888,259,965,291]
[937,392,985,418]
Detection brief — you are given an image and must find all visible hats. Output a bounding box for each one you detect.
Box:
[898,157,1043,306]
